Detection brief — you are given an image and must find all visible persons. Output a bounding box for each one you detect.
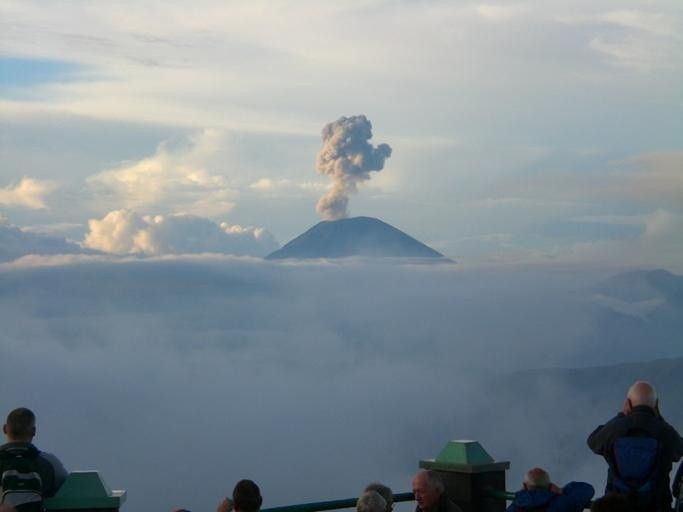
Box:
[0,407,68,511]
[364,484,393,512]
[356,491,387,512]
[218,478,264,512]
[506,467,595,511]
[410,466,460,512]
[586,380,681,510]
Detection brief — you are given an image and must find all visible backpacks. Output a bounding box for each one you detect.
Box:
[0,444,48,512]
